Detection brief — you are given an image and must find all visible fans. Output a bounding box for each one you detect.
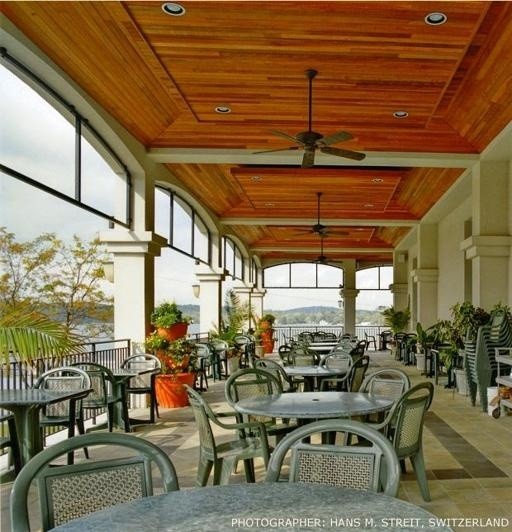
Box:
[305,239,344,266]
[293,192,349,238]
[252,68,366,167]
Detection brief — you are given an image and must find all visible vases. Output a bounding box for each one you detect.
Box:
[261,339,274,354]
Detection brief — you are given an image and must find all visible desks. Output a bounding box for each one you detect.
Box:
[85,367,158,432]
[309,342,337,347]
[307,346,342,354]
[0,387,94,484]
[281,365,347,393]
[47,481,455,532]
[236,391,395,448]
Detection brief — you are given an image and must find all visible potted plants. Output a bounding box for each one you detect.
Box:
[256,324,274,340]
[146,338,194,409]
[260,315,274,329]
[150,302,189,341]
[145,333,191,370]
[424,299,490,395]
[407,323,431,370]
[208,318,241,375]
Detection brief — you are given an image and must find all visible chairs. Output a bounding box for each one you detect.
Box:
[200,342,220,382]
[339,346,364,390]
[32,366,90,468]
[253,358,298,424]
[234,336,250,367]
[0,414,22,477]
[224,367,285,483]
[356,340,366,347]
[279,345,291,361]
[282,331,294,351]
[183,383,270,487]
[363,329,376,350]
[212,339,228,376]
[192,344,209,391]
[341,382,434,501]
[119,353,162,424]
[62,361,130,432]
[356,368,410,472]
[286,348,319,392]
[298,331,336,342]
[9,432,179,532]
[464,307,512,412]
[263,418,401,498]
[325,343,352,387]
[319,356,369,392]
[338,336,352,344]
[317,351,354,392]
